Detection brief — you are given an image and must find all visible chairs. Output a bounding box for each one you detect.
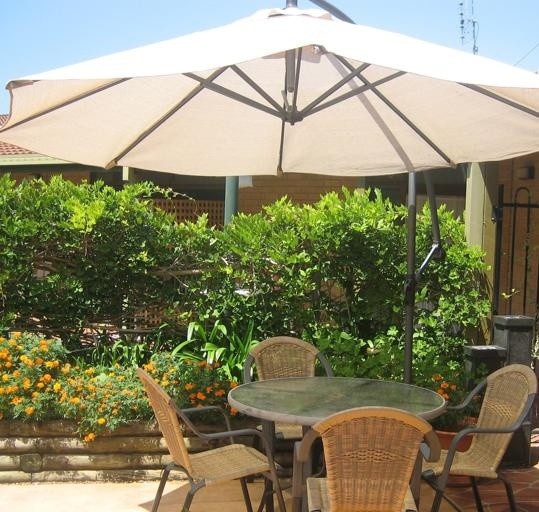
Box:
[421,363,539,512]
[243,335,335,483]
[137,366,287,512]
[288,406,441,512]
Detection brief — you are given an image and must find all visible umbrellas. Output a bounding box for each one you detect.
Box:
[1,0,539,383]
